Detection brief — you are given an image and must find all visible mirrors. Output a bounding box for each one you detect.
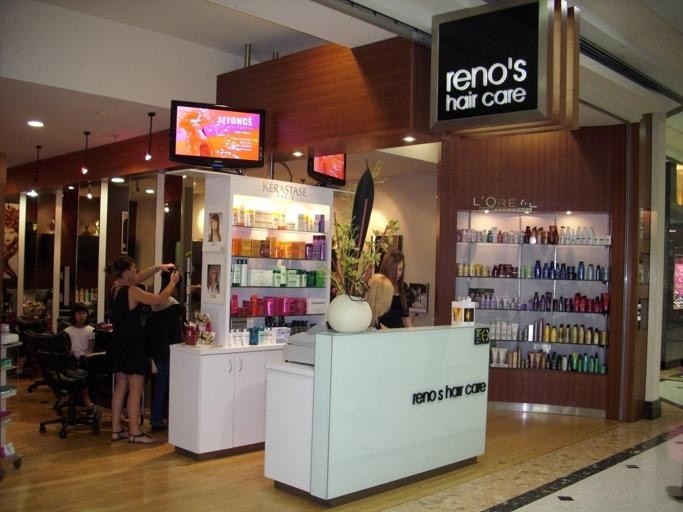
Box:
[58,179,101,333]
[105,171,158,319]
[22,186,57,333]
[161,172,206,325]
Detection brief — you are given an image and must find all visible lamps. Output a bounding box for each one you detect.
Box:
[81,131,91,175]
[144,112,155,161]
[86,179,93,200]
[26,145,41,197]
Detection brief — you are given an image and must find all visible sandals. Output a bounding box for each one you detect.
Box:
[129,433,156,444]
[111,429,129,440]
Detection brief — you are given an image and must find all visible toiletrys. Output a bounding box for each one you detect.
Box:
[639,255,644,282]
[636,299,642,330]
[640,208,644,239]
[229,205,327,347]
[456,223,612,374]
[75,287,94,305]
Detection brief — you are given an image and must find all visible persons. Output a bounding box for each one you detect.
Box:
[149,271,183,432]
[208,214,221,242]
[207,267,219,293]
[376,249,414,330]
[64,301,96,408]
[104,255,180,444]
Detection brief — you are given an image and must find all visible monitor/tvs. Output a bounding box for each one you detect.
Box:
[169,100,267,174]
[79,236,99,266]
[307,146,347,191]
[192,240,202,268]
[41,234,54,265]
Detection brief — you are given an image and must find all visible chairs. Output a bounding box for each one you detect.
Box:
[32,333,100,439]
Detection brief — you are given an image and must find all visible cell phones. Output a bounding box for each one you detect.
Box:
[171,268,177,273]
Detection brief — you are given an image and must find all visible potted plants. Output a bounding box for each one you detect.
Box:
[317,210,399,333]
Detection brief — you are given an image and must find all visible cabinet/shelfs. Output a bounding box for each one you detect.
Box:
[167,345,267,455]
[0,338,24,466]
[203,174,331,345]
[454,210,612,375]
